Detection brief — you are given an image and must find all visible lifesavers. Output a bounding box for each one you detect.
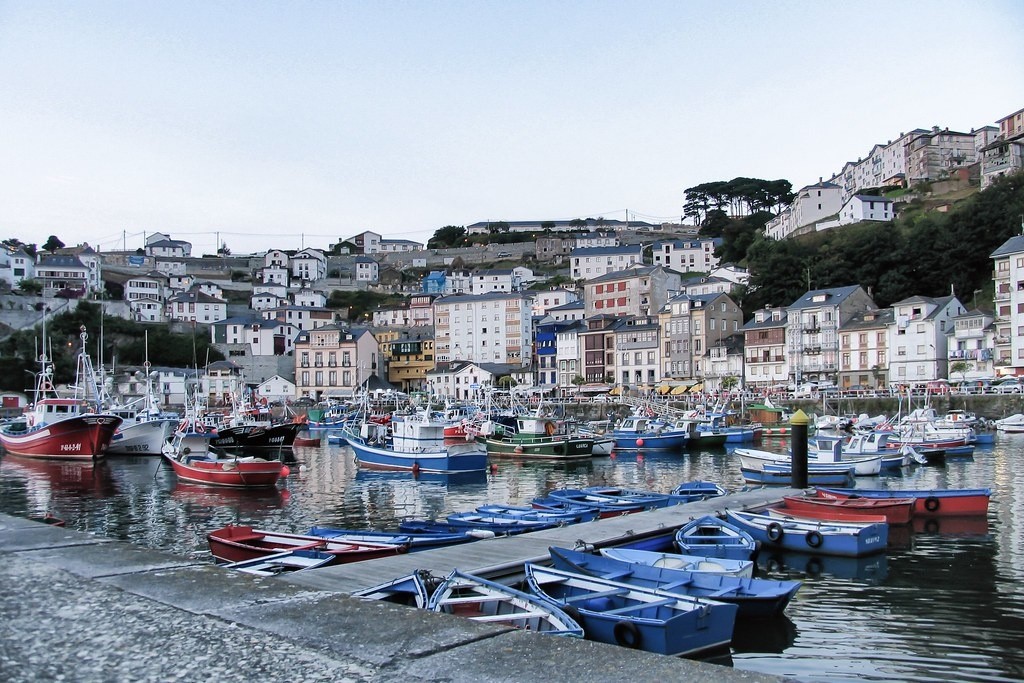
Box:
[875,424,893,431]
[672,528,678,540]
[648,408,654,417]
[560,605,580,624]
[751,538,761,560]
[806,558,824,577]
[179,420,189,432]
[29,415,34,426]
[924,496,940,513]
[195,420,206,434]
[848,494,859,499]
[806,530,824,550]
[767,521,783,542]
[691,412,697,417]
[40,382,52,390]
[766,555,784,572]
[671,541,682,554]
[924,517,939,535]
[614,620,644,649]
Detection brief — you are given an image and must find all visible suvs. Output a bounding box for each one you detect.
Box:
[789,385,813,398]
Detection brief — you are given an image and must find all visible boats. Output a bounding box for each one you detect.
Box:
[177,347,518,451]
[308,484,725,555]
[725,510,890,559]
[162,416,283,489]
[463,392,764,459]
[733,406,1024,487]
[676,516,756,560]
[76,325,182,456]
[0,321,123,463]
[784,485,992,524]
[657,383,704,395]
[548,547,805,626]
[343,413,489,474]
[206,520,406,578]
[523,564,737,659]
[353,567,585,639]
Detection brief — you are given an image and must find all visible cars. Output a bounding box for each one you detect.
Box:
[189,392,459,407]
[821,378,1024,397]
